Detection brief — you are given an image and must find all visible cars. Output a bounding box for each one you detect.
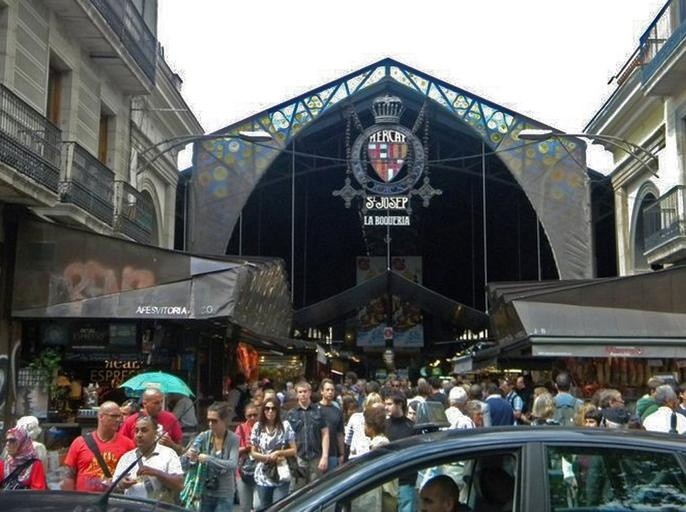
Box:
[0,489,209,511]
[255,422,686,512]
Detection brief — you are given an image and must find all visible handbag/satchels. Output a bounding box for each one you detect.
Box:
[261,426,307,483]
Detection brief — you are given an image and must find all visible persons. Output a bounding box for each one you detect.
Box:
[1,372,685,510]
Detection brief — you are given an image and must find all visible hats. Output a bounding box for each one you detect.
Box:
[448,385,468,404]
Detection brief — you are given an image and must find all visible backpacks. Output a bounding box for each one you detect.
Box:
[234,386,252,416]
[239,456,259,485]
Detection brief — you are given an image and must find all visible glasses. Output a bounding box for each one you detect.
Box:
[245,413,258,417]
[103,414,122,419]
[206,418,220,425]
[263,406,279,411]
[391,384,399,387]
[4,437,19,444]
